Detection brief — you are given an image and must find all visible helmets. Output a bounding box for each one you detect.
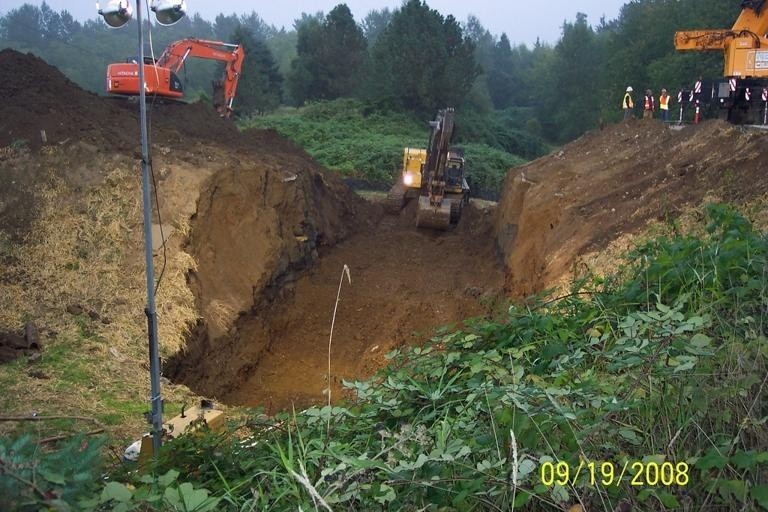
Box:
[625,86,634,92]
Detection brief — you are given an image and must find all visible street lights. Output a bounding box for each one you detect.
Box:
[95,0,190,485]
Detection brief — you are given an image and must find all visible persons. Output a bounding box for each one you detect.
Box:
[642,88,655,118]
[658,87,672,120]
[622,86,634,120]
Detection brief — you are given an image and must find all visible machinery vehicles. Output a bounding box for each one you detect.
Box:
[101,37,245,119]
[386,107,472,230]
[674,0,767,130]
